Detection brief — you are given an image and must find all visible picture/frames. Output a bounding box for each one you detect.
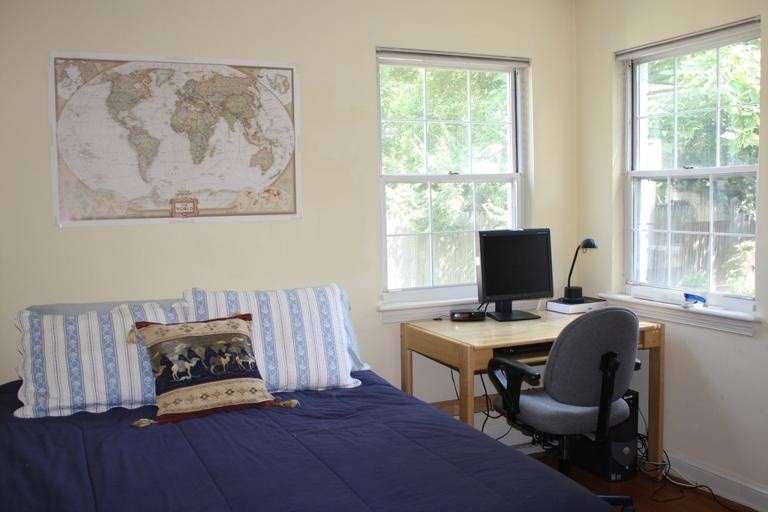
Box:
[48,50,306,229]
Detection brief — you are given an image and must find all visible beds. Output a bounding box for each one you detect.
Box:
[0,368,621,511]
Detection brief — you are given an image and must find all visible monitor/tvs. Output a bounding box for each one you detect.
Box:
[477,227,555,323]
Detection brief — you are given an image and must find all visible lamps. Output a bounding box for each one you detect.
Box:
[557,237,599,304]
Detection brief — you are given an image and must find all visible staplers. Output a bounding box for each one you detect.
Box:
[682,293,706,308]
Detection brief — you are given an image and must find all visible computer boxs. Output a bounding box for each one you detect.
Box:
[603,389,640,483]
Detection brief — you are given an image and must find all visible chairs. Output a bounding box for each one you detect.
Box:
[487,306,643,509]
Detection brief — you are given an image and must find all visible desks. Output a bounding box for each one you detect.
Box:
[399,308,666,483]
